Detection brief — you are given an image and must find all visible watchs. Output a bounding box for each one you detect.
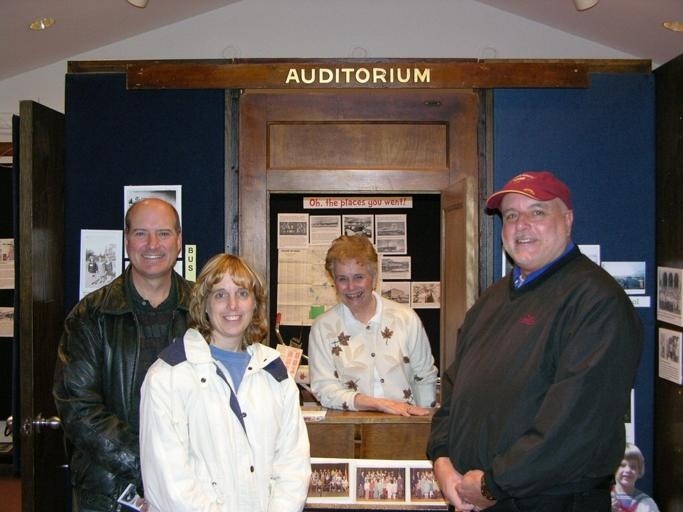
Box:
[478,472,497,501]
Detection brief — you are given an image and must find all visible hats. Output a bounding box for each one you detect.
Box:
[482,170,573,214]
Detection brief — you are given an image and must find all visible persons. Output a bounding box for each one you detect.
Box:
[611,440,661,512]
[306,467,442,500]
[423,287,434,302]
[50,197,198,511]
[136,254,311,512]
[307,233,439,418]
[424,169,647,509]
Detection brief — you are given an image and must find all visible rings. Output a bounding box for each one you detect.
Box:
[406,405,412,413]
